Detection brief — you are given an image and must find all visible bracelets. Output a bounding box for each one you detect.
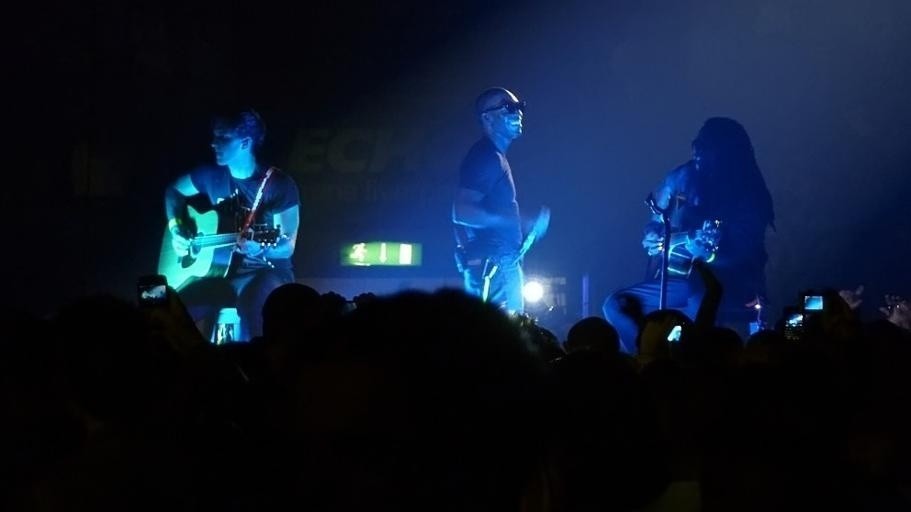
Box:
[250,243,265,259]
[167,215,185,231]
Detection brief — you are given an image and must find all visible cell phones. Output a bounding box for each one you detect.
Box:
[784,312,807,342]
[797,294,828,314]
[135,274,171,315]
[663,322,686,353]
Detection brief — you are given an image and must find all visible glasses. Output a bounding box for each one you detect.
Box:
[484,99,527,114]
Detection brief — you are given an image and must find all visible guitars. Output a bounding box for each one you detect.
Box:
[647,197,727,280]
[156,205,281,291]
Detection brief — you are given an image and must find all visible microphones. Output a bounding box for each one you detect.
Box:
[256,165,267,179]
[520,201,550,252]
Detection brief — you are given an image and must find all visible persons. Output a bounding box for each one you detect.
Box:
[449,85,550,319]
[600,113,777,358]
[2,282,911,512]
[162,103,299,345]
